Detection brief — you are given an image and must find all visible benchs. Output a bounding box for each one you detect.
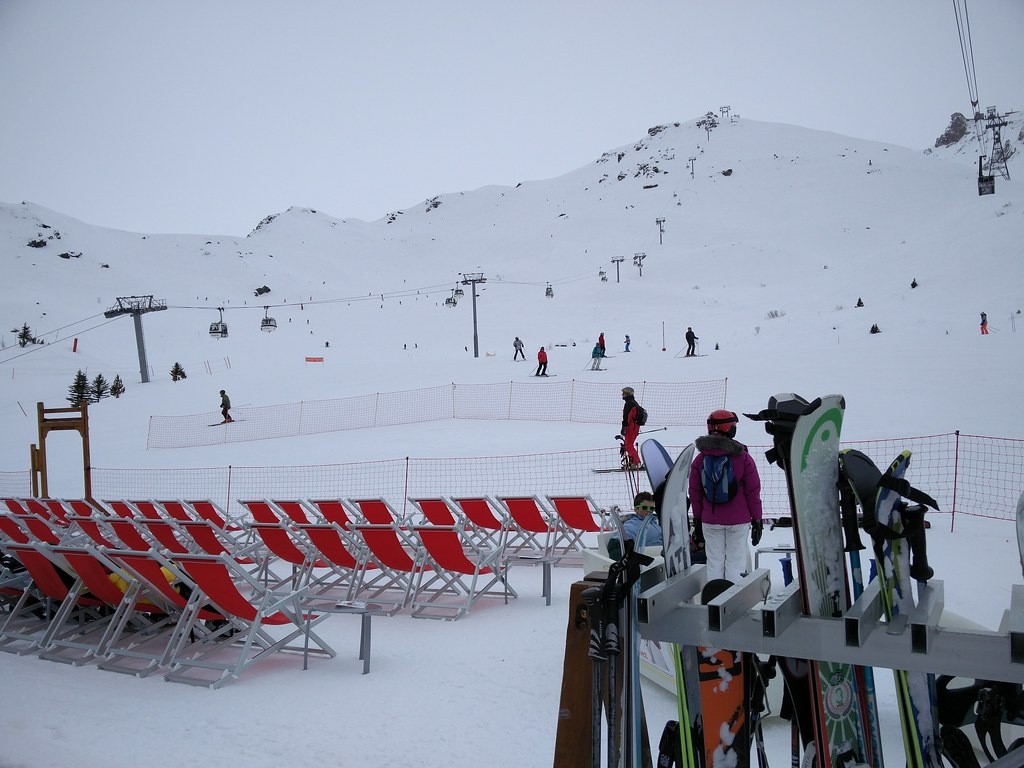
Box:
[580,547,677,695]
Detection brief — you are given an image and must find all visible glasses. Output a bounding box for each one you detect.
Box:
[637,505,655,512]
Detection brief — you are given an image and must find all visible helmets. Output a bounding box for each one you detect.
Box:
[707,409,739,438]
[220,390,225,394]
[621,387,634,395]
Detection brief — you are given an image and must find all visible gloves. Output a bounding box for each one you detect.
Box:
[693,518,705,543]
[751,520,763,546]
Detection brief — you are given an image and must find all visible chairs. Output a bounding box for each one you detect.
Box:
[597,529,618,558]
[0,493,618,691]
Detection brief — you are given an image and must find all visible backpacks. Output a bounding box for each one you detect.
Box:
[630,398,647,426]
[696,459,738,513]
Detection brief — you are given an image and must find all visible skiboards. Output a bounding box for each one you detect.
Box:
[530,375,557,378]
[590,468,647,473]
[208,419,246,426]
[637,436,705,768]
[768,389,865,768]
[836,445,944,768]
[678,354,708,358]
[587,369,607,371]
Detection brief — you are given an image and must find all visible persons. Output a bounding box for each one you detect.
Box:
[599,333,607,357]
[610,494,666,546]
[536,347,547,376]
[619,387,642,468]
[513,336,525,361]
[686,326,699,356]
[688,410,765,586]
[591,342,601,370]
[624,334,630,351]
[980,311,989,334]
[219,390,232,422]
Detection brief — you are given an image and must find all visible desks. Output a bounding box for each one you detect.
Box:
[754,547,796,570]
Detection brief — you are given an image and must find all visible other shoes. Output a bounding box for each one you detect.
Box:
[541,373,547,375]
[535,374,541,376]
[622,463,642,471]
[596,368,601,371]
[686,355,691,357]
[691,354,697,356]
